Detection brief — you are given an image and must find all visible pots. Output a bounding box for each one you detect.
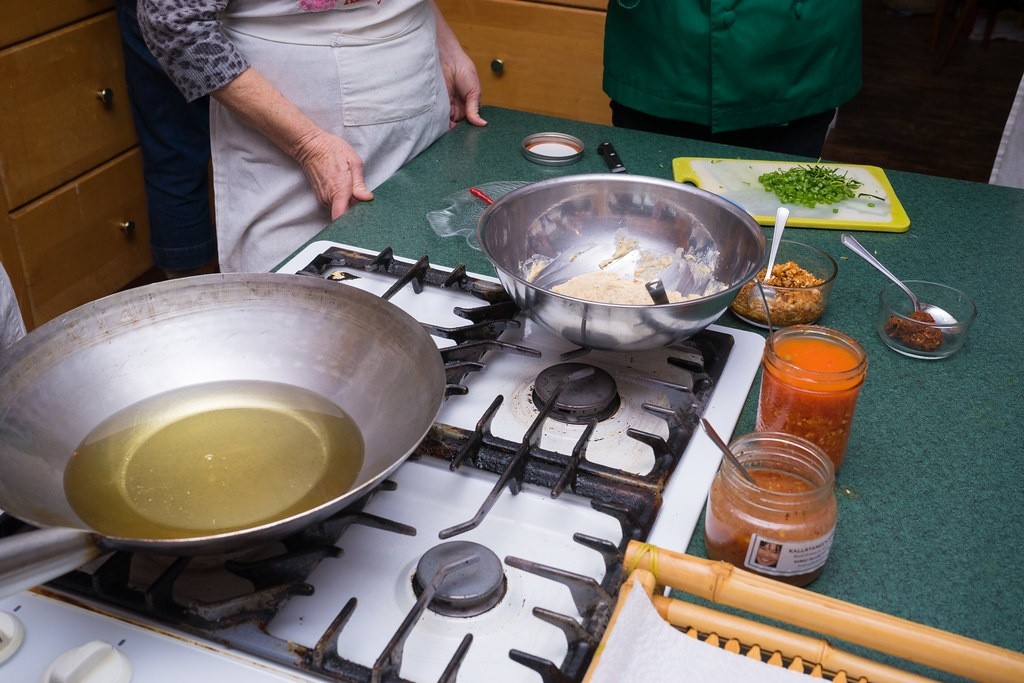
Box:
[2,273,450,602]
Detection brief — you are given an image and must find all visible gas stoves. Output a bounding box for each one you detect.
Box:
[4,240,765,682]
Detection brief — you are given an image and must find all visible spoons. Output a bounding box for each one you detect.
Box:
[748,207,789,315]
[841,233,963,333]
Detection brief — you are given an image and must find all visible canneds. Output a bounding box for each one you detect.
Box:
[703,432,838,587]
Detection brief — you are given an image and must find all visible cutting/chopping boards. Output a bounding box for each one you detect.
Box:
[672,157,911,232]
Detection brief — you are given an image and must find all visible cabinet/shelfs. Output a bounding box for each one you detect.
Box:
[1,0,156,325]
[428,0,617,124]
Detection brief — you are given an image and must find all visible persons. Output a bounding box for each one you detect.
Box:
[137,0,487,272]
[603,0,867,160]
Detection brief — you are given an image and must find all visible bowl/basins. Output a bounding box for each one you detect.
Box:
[729,238,837,331]
[879,280,978,359]
[479,174,768,350]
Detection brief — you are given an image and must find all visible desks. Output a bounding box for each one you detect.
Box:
[1,107,1024,683]
